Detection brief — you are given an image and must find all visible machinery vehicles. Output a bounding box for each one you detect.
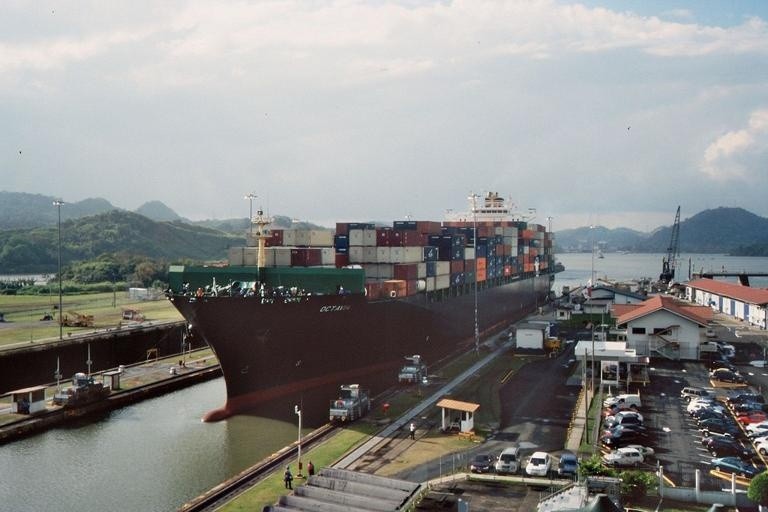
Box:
[59,311,98,329]
[326,382,371,425]
[395,353,429,384]
[120,304,145,321]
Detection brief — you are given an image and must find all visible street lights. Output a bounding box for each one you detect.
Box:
[586,321,610,399]
[293,403,304,479]
[546,215,553,295]
[589,224,594,285]
[112,272,116,308]
[51,201,66,339]
[243,193,259,235]
[466,187,482,354]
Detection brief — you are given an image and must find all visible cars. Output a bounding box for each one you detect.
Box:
[679,342,768,478]
[705,327,716,337]
[470,443,656,480]
[599,390,647,447]
[72,371,87,384]
[52,386,79,404]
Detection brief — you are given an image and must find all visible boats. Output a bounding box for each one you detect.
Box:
[598,253,604,259]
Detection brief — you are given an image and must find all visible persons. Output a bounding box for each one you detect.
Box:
[283,464,294,490]
[182,279,345,298]
[306,460,315,476]
[337,396,343,409]
[384,400,390,416]
[409,422,416,440]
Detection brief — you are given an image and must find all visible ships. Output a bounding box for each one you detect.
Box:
[162,184,569,426]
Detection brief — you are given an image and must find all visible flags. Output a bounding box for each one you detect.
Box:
[581,287,592,300]
